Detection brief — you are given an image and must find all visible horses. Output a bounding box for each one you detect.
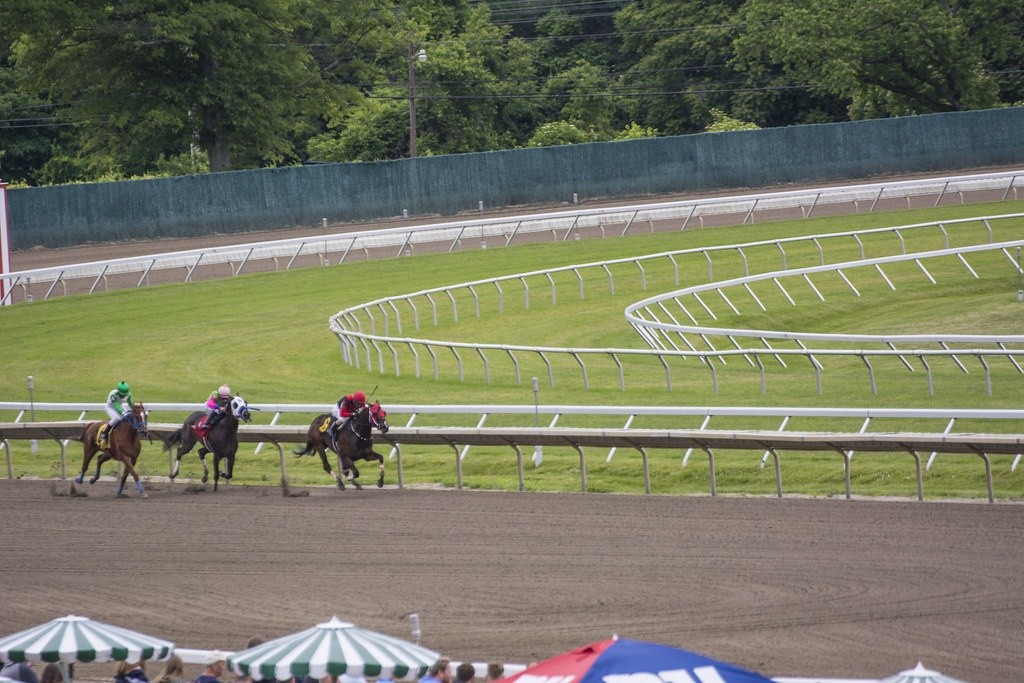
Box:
[162,391,251,493]
[71,399,151,499]
[292,399,389,490]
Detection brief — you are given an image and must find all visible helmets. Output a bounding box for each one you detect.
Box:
[117,381,129,394]
[219,385,229,399]
[352,391,366,404]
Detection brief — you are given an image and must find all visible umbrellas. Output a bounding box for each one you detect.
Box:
[495,633,779,683]
[0,614,176,679]
[225,616,441,679]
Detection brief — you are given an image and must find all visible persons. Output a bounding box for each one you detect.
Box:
[113,660,149,683]
[201,385,230,428]
[193,654,227,683]
[418,657,451,683]
[150,653,189,683]
[102,381,135,437]
[325,391,366,437]
[253,675,396,681]
[0,660,65,683]
[454,663,476,683]
[486,662,504,682]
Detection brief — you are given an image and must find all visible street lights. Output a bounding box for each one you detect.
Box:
[407,39,427,158]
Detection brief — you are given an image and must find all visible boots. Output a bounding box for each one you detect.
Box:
[201,410,216,428]
[328,421,340,435]
[98,423,113,441]
